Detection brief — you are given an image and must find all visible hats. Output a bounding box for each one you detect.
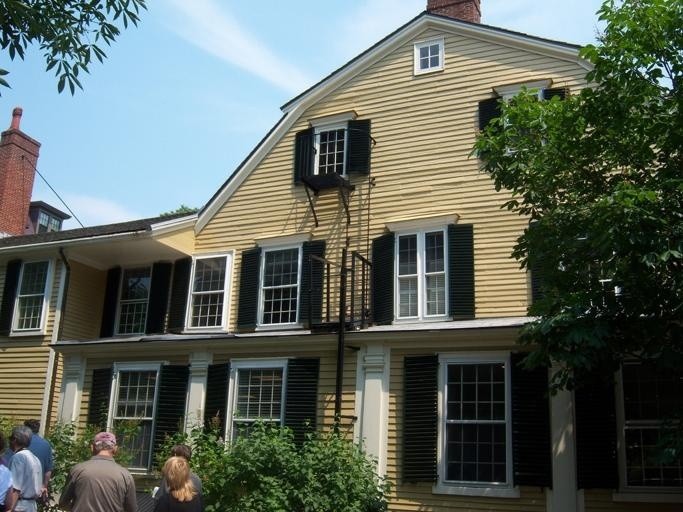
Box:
[95,432,116,444]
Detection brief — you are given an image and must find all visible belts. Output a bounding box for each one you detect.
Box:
[18,498,34,500]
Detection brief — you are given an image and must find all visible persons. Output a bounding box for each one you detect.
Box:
[153,456,203,511]
[0,418,54,512]
[153,444,202,507]
[59,431,139,512]
